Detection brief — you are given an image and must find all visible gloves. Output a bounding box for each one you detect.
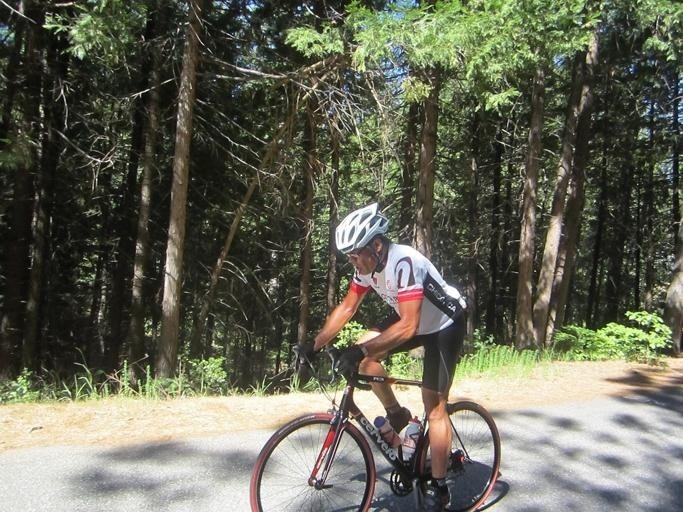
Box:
[335,346,368,379]
[295,338,315,373]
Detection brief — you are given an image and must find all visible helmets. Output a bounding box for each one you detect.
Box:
[335,202,389,255]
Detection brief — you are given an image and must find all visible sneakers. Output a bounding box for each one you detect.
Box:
[422,479,450,512]
[386,407,410,433]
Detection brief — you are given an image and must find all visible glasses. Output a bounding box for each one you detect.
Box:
[345,248,362,258]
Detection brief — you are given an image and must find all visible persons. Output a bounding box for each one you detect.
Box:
[289,202,469,510]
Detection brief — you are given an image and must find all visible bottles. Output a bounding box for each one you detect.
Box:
[400,414,420,462]
[373,415,401,447]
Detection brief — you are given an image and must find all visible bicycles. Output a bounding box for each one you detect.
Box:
[248,338,499,511]
